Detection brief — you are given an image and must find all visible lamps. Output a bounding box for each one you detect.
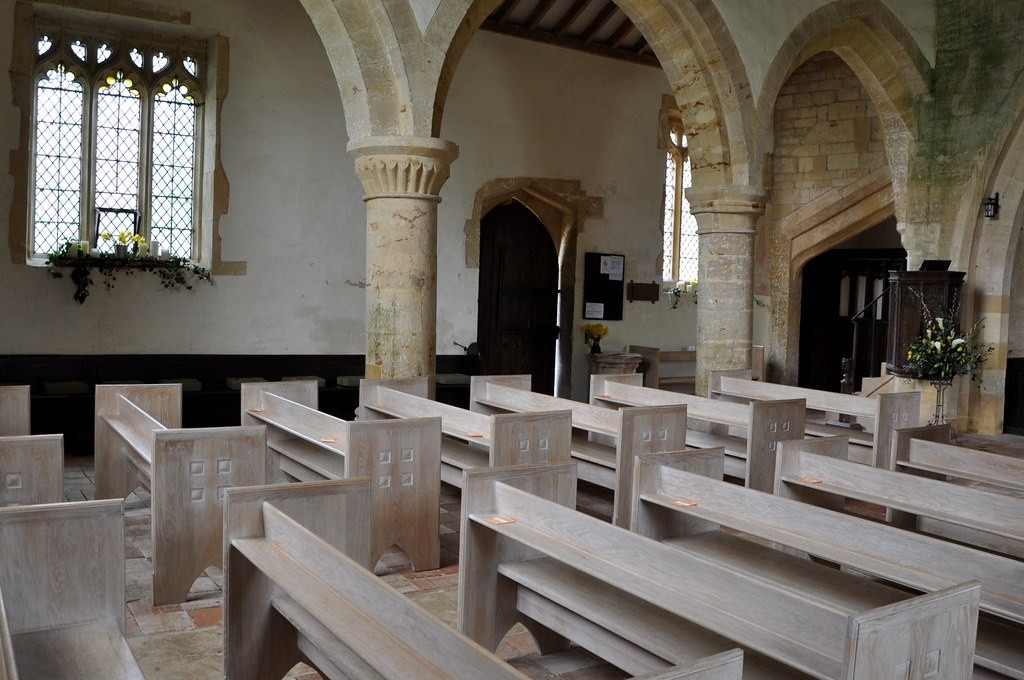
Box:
[984,192,1000,218]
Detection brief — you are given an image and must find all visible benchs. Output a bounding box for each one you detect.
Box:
[2,370,1022,678]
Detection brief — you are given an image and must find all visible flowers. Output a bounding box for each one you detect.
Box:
[905,286,997,392]
[100,232,146,245]
[581,322,608,339]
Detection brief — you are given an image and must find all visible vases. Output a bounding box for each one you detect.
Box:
[113,245,128,258]
[590,333,601,354]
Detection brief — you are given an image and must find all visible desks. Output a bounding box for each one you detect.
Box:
[628,343,766,391]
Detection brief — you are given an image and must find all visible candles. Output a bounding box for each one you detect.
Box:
[161,250,169,261]
[138,243,147,258]
[69,240,78,256]
[81,241,90,254]
[149,240,158,259]
